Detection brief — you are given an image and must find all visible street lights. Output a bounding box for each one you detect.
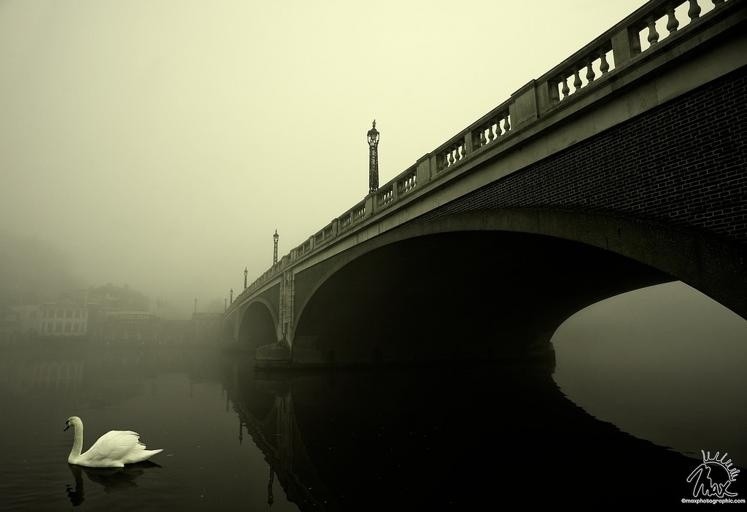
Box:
[223,266,248,313]
[272,228,280,266]
[365,118,380,193]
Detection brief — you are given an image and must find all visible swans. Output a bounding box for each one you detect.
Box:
[63,415,164,468]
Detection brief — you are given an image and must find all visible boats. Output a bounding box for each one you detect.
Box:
[255,331,290,372]
